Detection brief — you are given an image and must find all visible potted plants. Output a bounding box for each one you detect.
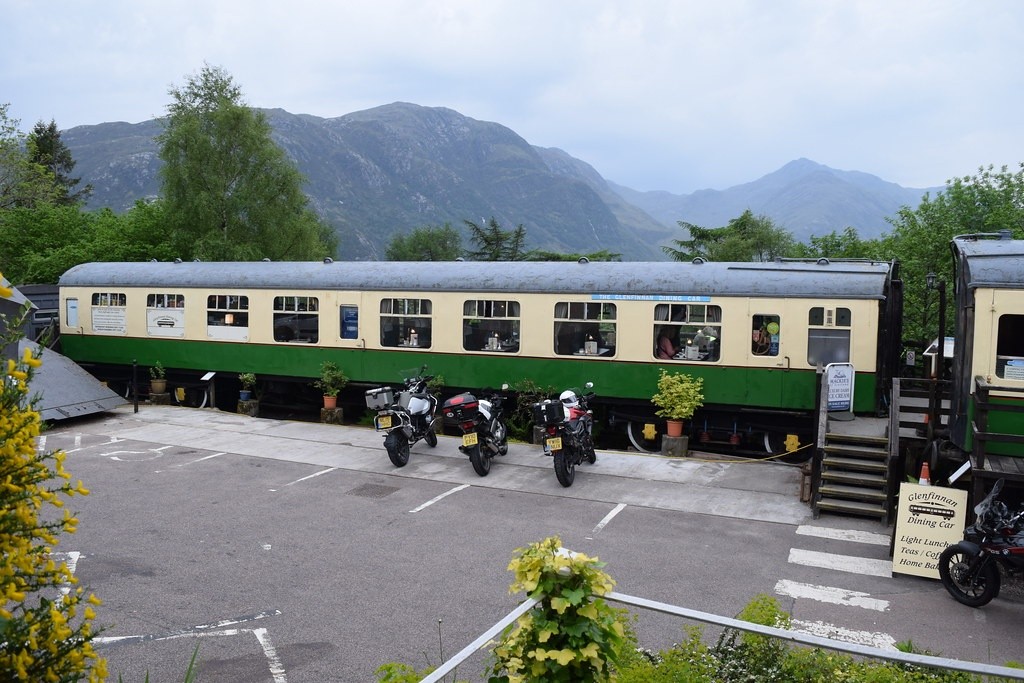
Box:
[651,368,704,437]
[239,373,257,401]
[150,360,166,394]
[314,361,350,410]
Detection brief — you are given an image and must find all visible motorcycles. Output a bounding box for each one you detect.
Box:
[365,364,437,468]
[938,479,1024,608]
[443,384,510,477]
[531,382,597,489]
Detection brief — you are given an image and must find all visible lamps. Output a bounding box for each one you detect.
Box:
[407,328,415,345]
[225,314,233,324]
[489,330,499,351]
[585,333,593,354]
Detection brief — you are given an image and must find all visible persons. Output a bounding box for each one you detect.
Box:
[656,326,680,359]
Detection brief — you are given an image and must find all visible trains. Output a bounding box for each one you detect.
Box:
[948,230,1024,459]
[58,260,905,416]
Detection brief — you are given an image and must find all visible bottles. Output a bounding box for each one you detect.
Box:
[1007,360,1024,366]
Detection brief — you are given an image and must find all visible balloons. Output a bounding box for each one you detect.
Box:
[767,322,779,335]
